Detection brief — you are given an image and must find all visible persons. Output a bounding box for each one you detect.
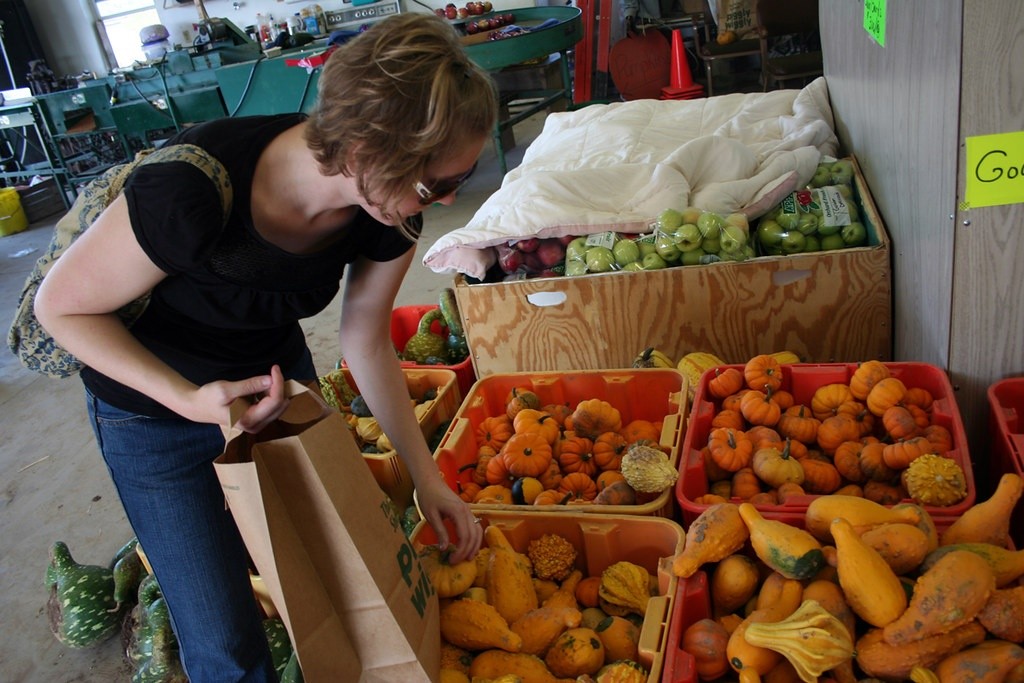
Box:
[33,13,499,683]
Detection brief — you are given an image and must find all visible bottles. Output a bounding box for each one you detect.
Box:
[256,13,274,50]
[269,17,280,42]
[245,25,260,42]
[294,12,305,32]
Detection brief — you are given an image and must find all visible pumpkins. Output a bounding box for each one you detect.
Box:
[416,522,659,683]
[671,474,1024,683]
[319,357,444,454]
[453,387,678,507]
[631,345,967,510]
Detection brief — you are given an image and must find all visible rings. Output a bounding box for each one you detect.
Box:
[473,518,482,524]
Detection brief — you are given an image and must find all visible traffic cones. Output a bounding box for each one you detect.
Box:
[658,29,705,101]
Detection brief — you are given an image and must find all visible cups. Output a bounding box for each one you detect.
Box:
[285,15,303,35]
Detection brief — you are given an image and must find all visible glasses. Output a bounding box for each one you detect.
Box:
[411,161,479,205]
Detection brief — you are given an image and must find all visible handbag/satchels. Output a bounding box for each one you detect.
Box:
[213,380,440,683]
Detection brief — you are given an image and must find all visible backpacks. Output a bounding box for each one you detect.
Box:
[7,144,233,379]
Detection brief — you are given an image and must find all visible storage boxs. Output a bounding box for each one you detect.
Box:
[317,156,1024,683]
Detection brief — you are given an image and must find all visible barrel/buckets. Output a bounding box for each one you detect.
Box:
[0,187,29,237]
[14,176,70,223]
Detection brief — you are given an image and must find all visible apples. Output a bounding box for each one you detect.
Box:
[497,160,867,279]
[434,1,525,40]
